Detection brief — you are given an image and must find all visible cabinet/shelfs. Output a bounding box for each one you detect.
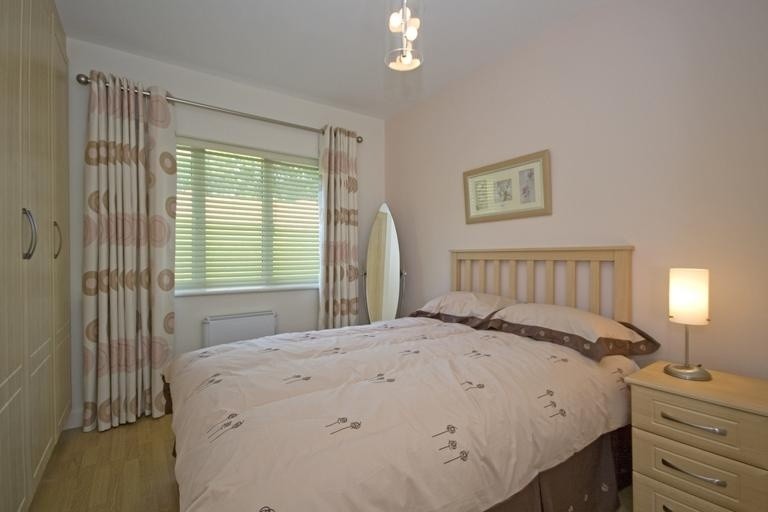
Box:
[0,0,75,512]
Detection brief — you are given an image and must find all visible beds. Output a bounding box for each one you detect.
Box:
[162,244,641,512]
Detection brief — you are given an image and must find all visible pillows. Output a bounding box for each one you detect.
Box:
[410,290,518,330]
[484,302,661,363]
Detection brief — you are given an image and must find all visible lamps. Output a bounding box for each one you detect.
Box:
[661,267,712,382]
[383,0,424,71]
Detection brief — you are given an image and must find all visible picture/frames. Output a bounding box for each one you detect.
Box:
[461,149,552,224]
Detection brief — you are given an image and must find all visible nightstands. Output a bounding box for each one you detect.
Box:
[622,362,766,512]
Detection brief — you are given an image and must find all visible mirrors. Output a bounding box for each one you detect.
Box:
[362,204,406,324]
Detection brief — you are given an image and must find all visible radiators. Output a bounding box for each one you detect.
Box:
[202,310,275,348]
